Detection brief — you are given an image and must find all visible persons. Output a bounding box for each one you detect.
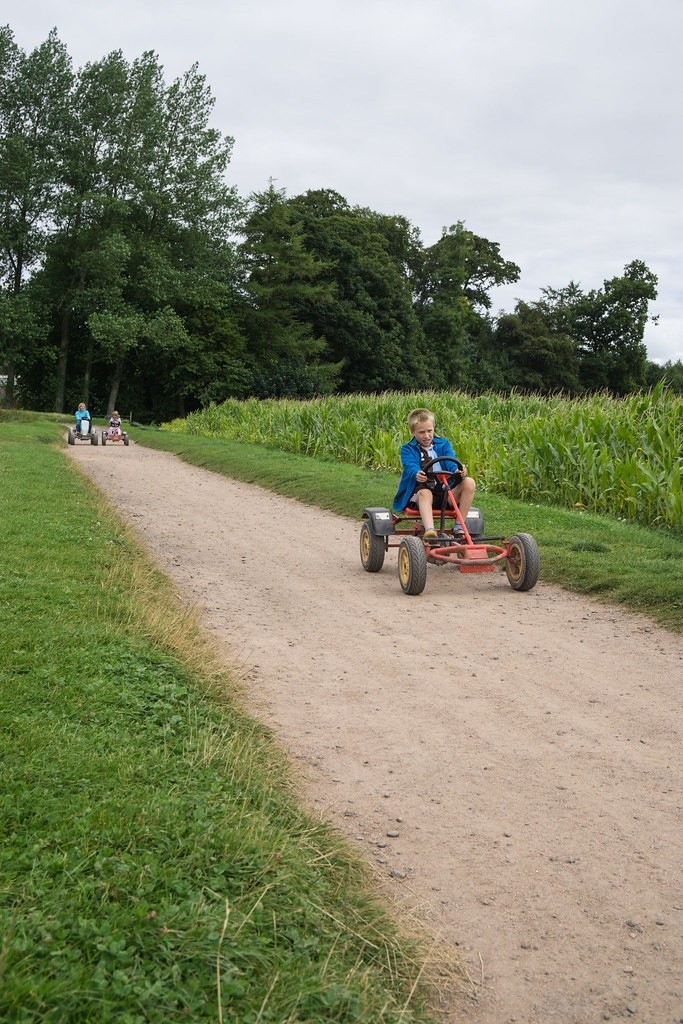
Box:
[390,408,476,551]
[109,411,122,437]
[75,402,92,438]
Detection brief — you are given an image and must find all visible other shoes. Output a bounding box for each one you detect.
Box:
[451,528,467,543]
[422,530,439,543]
